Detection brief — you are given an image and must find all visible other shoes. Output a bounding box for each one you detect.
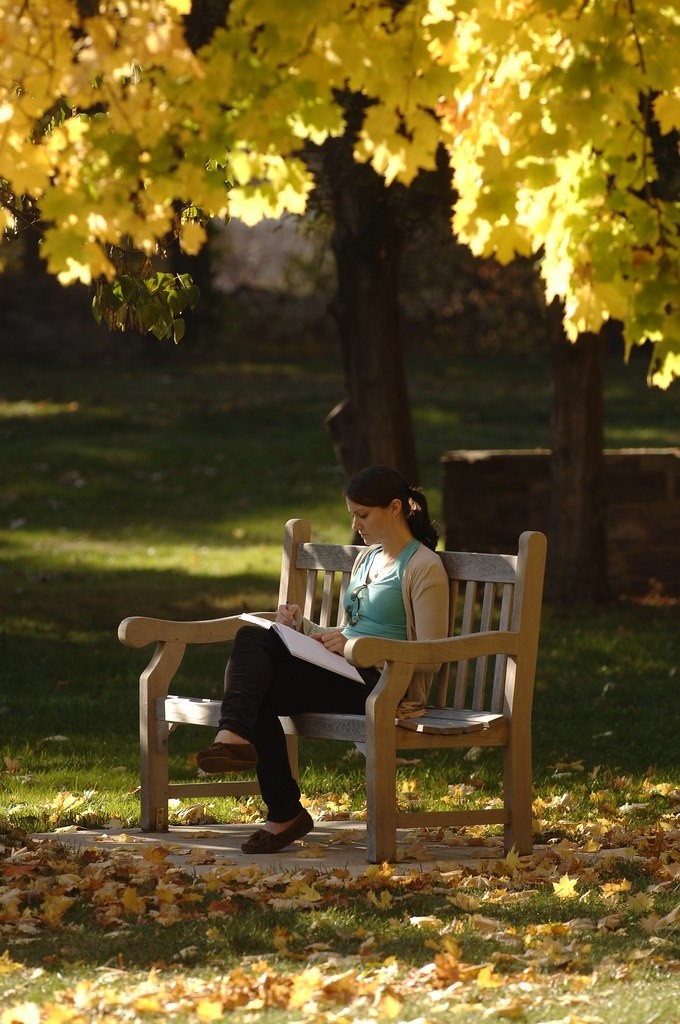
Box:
[241,808,315,855]
[197,741,259,773]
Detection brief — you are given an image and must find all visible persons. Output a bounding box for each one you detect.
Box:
[195,463,449,853]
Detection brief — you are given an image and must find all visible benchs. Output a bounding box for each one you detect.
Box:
[117,517,548,863]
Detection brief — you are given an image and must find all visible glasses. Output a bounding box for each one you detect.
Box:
[344,584,367,626]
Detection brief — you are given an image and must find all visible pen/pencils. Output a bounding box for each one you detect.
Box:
[286,600,297,631]
